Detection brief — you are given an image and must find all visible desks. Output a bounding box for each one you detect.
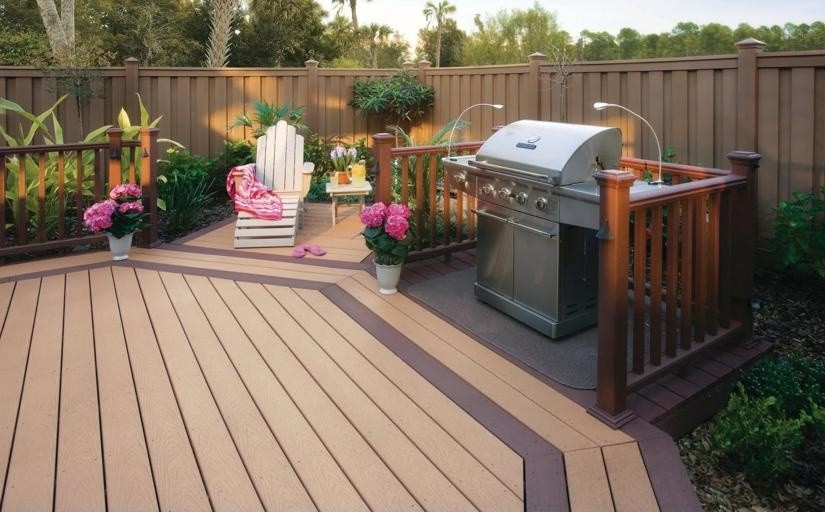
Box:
[326,180,372,226]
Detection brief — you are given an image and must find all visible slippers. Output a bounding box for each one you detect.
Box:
[293,244,326,258]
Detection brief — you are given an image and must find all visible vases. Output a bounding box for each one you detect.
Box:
[335,172,351,184]
[373,261,402,294]
[105,232,133,261]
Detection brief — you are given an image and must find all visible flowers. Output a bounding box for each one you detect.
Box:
[82,181,154,240]
[329,145,358,171]
[351,201,415,265]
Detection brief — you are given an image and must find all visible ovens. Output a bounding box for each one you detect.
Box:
[442,118,671,338]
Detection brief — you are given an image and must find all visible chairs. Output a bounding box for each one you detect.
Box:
[232,120,315,251]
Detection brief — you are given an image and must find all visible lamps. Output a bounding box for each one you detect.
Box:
[447,102,504,159]
[593,100,664,186]
